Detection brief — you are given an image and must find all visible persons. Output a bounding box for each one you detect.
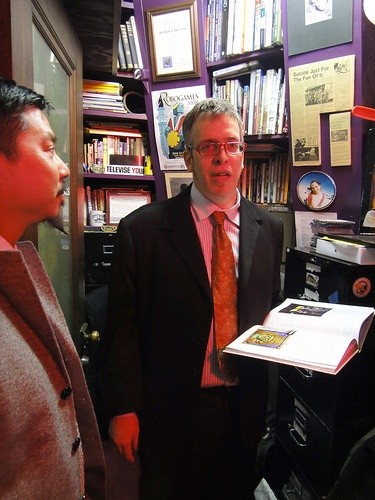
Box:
[299,180,334,209]
[100,98,284,500]
[0,81,107,500]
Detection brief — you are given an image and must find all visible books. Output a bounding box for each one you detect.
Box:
[236,143,291,206]
[83,121,147,175]
[81,79,126,114]
[212,59,286,136]
[204,0,283,63]
[84,186,151,226]
[116,15,144,72]
[222,298,375,375]
[310,219,356,253]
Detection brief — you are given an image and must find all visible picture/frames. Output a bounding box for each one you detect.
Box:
[165,173,193,199]
[296,170,337,211]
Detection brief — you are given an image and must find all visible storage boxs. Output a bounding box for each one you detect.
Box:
[316,239,375,265]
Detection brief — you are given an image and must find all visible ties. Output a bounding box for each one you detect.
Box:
[210,210,239,385]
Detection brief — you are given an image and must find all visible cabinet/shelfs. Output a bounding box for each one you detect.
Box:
[270,249,375,500]
[82,0,289,289]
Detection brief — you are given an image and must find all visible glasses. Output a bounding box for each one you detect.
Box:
[189,141,248,156]
